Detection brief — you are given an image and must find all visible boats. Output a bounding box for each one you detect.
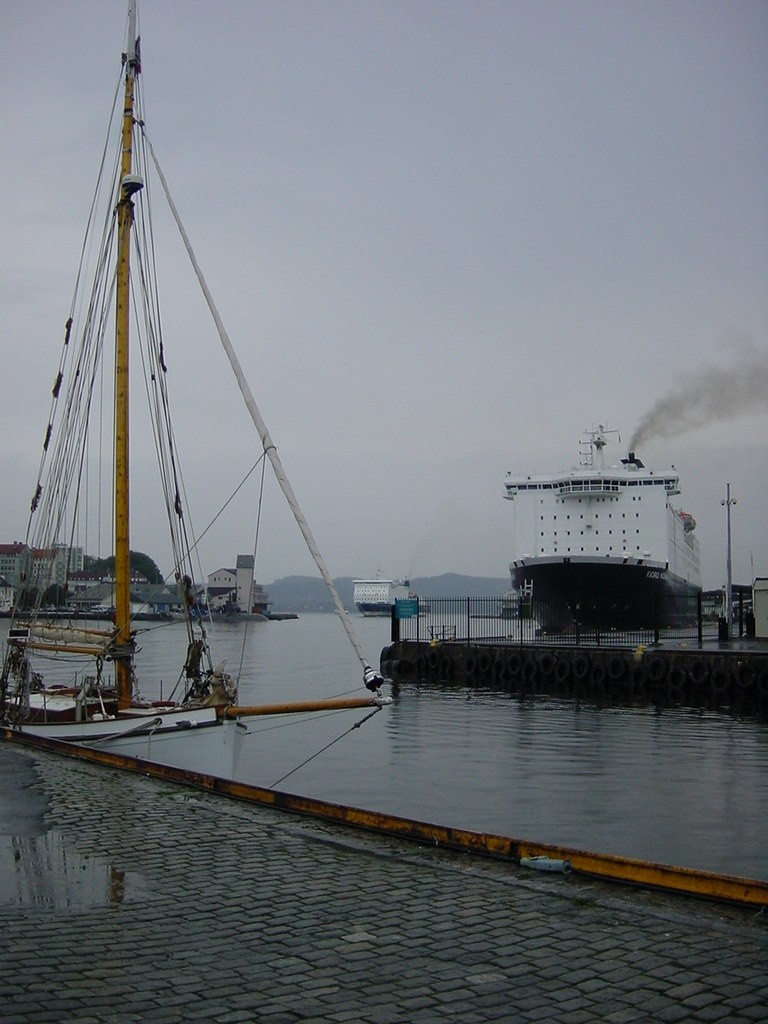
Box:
[496,422,702,642]
[350,567,429,618]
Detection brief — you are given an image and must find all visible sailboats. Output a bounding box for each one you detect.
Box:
[0,2,397,793]
